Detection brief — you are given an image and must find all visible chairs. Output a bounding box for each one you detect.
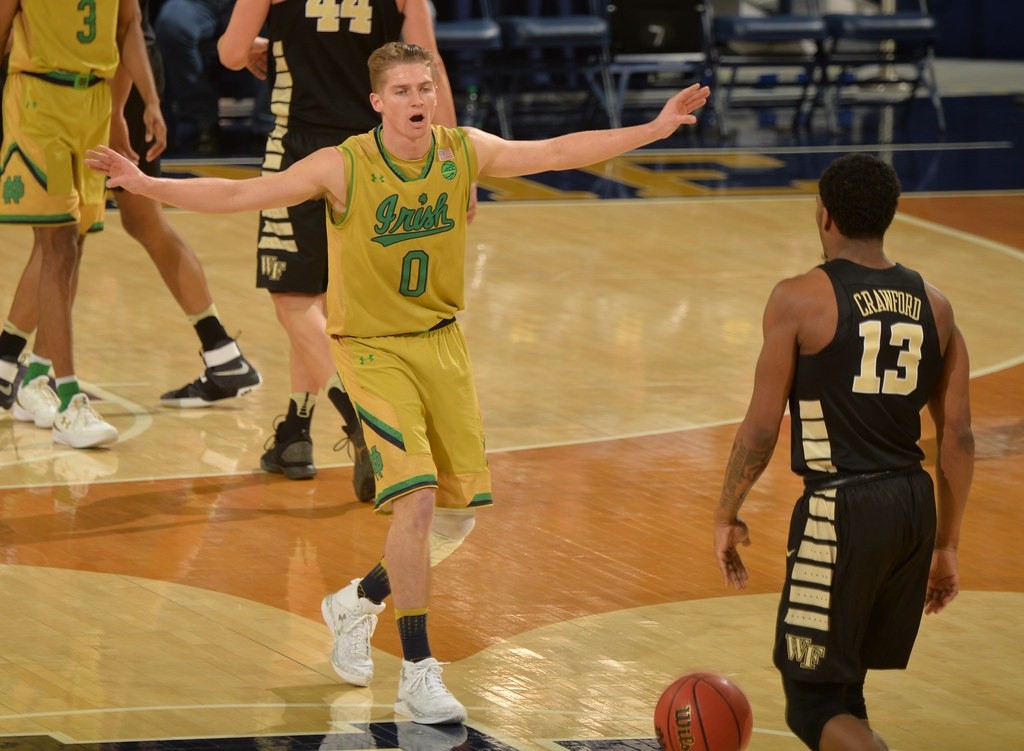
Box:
[433,1,947,142]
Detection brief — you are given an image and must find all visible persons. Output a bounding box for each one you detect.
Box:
[218,0,477,503]
[712,154,976,751]
[0,0,260,449]
[83,43,710,726]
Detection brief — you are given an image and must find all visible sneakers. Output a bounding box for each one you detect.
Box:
[393,657,467,724]
[260,414,317,479]
[51,394,119,448]
[11,376,61,428]
[321,577,386,687]
[0,359,19,409]
[333,421,375,502]
[158,338,263,408]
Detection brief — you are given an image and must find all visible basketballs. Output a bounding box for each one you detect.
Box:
[651,670,754,751]
